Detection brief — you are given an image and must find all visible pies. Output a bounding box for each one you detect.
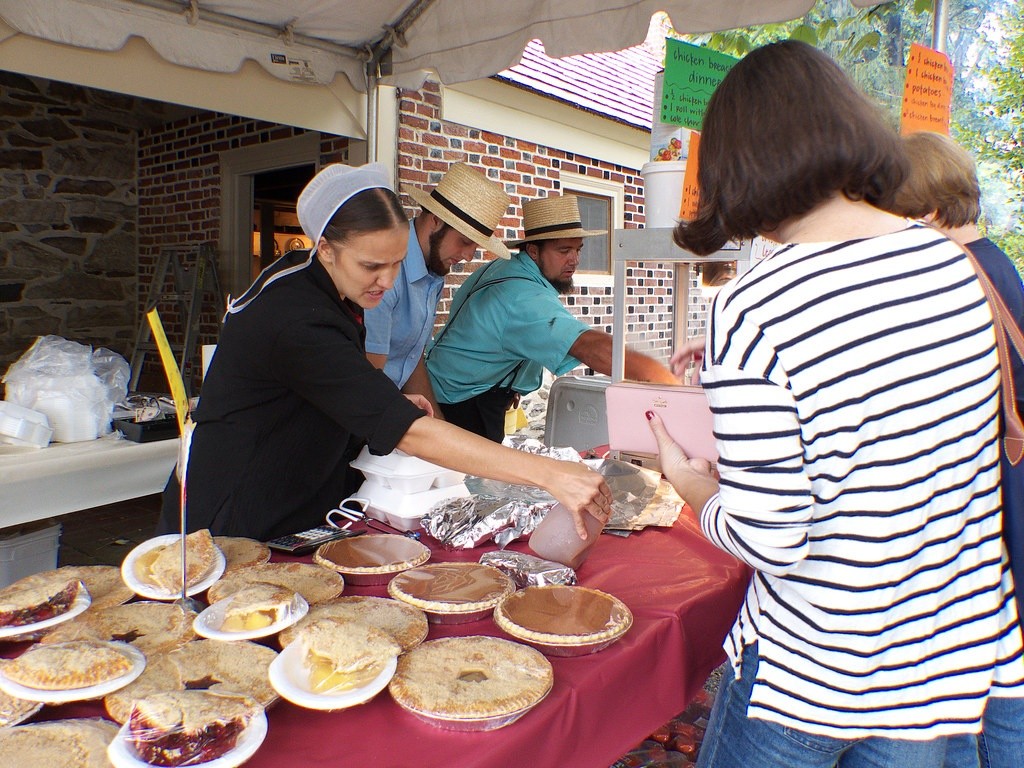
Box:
[0,530,634,768]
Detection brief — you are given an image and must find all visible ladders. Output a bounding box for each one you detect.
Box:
[130,239,224,396]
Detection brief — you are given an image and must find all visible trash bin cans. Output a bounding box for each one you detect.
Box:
[0,520,62,589]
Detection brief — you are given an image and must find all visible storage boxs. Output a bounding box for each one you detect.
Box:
[0,518,62,588]
[113,416,179,443]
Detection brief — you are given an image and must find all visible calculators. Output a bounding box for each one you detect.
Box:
[267,526,367,553]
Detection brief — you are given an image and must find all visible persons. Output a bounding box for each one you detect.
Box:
[643,38,1024,768]
[889,129,1024,768]
[151,161,620,539]
[363,154,511,422]
[423,193,680,444]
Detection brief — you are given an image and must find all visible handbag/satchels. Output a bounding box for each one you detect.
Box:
[916,220,1024,631]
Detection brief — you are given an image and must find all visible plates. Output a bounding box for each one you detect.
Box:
[1,533,634,768]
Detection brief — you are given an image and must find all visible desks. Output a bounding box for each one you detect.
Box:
[0,442,756,768]
[0,430,178,528]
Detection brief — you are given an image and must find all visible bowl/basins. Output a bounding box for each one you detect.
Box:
[349,445,474,534]
[0,374,100,448]
[528,502,612,569]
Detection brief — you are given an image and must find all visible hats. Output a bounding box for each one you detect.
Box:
[401,162,511,261]
[295,163,397,249]
[500,195,609,249]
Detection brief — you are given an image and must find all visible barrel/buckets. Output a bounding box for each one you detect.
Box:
[640,162,689,229]
